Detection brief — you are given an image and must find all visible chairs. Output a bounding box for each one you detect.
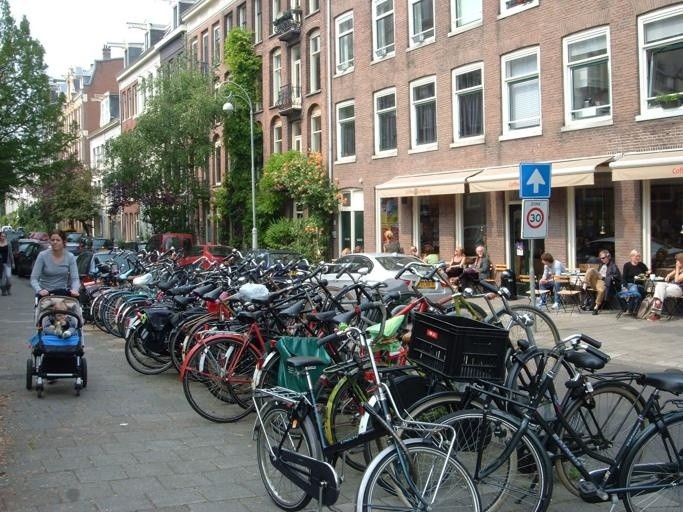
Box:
[466,257,507,294]
[518,263,682,320]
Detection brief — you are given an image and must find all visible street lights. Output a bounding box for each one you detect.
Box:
[217,81,258,249]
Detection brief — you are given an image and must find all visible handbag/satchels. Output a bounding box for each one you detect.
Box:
[581,297,608,311]
[139,309,178,356]
[637,296,661,319]
[277,335,331,393]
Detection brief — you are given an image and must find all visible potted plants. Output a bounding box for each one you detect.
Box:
[655,92,683,109]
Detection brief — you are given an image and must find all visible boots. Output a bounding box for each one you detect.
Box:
[2,285,12,296]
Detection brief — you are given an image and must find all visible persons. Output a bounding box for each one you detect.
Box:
[423,243,439,265]
[409,245,422,259]
[31,228,82,385]
[538,253,568,310]
[0,231,17,296]
[342,244,363,255]
[580,249,620,315]
[468,245,491,279]
[445,247,466,278]
[645,252,683,321]
[42,300,75,338]
[383,230,400,253]
[617,249,649,314]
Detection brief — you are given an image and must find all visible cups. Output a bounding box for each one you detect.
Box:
[649,274,656,280]
[575,268,580,274]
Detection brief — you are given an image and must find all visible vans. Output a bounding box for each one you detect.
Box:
[146,235,193,252]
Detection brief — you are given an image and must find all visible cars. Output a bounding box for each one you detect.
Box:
[2,225,111,278]
[73,250,150,320]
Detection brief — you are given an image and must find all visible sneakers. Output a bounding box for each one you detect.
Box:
[551,302,560,309]
[537,299,547,307]
[647,312,660,320]
[592,309,598,315]
[652,299,662,311]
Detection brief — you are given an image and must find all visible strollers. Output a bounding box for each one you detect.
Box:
[27,292,86,399]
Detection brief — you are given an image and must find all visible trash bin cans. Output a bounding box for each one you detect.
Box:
[499,269,518,301]
